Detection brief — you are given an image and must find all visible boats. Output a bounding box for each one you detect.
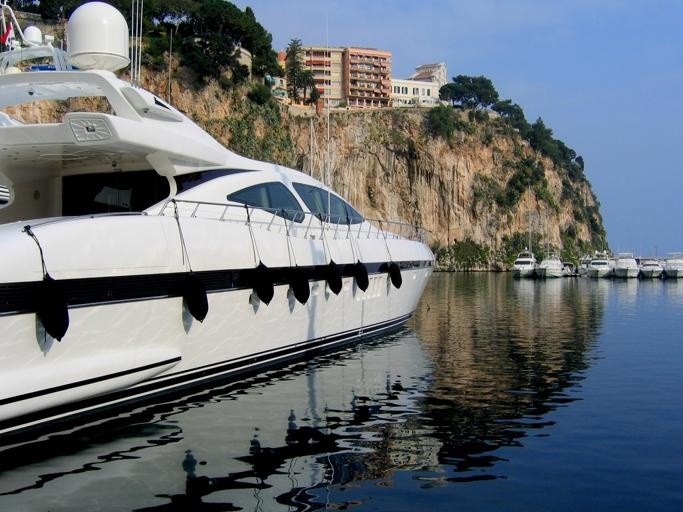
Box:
[511,252,683,280]
[1,1,437,433]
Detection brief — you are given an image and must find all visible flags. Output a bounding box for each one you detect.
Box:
[0,21,16,49]
